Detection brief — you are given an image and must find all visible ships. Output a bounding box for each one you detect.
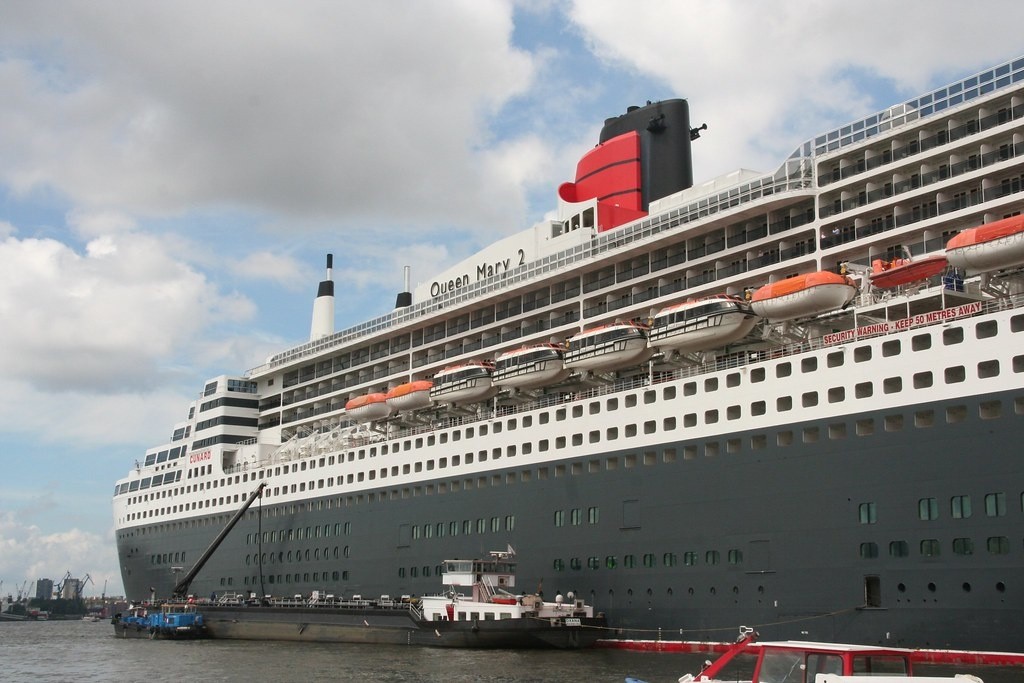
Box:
[113,48,1024,683]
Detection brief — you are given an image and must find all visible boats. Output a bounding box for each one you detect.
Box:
[345,392,391,424]
[646,291,760,356]
[112,606,212,642]
[866,255,950,289]
[0,606,50,622]
[561,319,653,376]
[386,380,433,412]
[490,341,572,393]
[944,212,1024,274]
[750,269,859,325]
[429,359,499,407]
[83,616,101,623]
[129,542,611,653]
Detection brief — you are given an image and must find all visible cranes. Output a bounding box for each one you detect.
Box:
[72,574,95,600]
[101,580,108,606]
[15,580,35,604]
[54,570,72,600]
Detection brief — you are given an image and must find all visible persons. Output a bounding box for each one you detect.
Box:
[210,592,215,601]
[193,592,198,600]
